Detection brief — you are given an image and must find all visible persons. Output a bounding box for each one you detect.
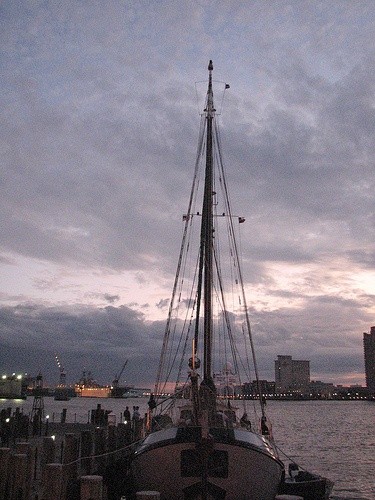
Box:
[132,406,136,419]
[134,406,141,419]
[123,406,131,423]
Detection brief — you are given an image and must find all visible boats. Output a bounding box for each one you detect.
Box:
[1,358,139,402]
[276,463,334,500]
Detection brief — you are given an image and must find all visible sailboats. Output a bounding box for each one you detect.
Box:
[123,60,287,500]
